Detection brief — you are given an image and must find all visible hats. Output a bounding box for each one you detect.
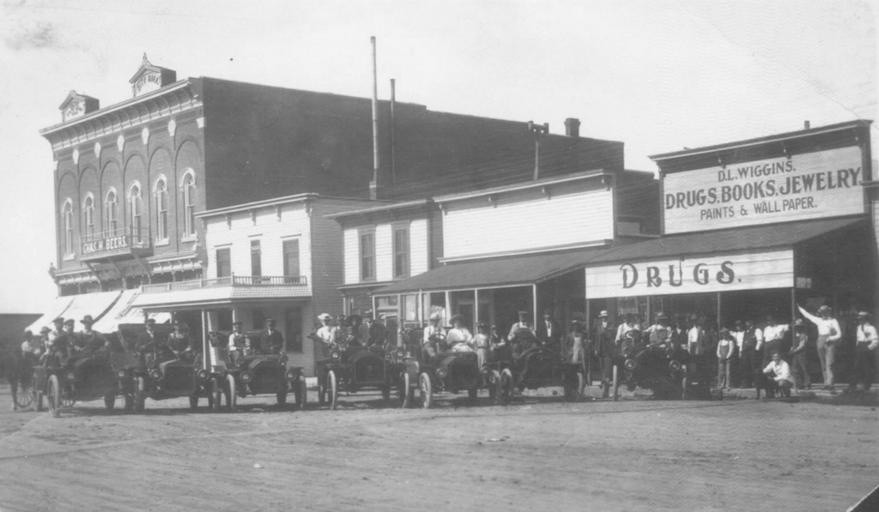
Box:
[449,314,463,324]
[233,320,243,325]
[40,318,73,333]
[429,313,440,320]
[598,310,608,318]
[80,316,93,322]
[544,309,553,313]
[317,311,374,322]
[655,312,668,320]
[518,309,529,314]
[856,312,868,320]
[817,305,832,314]
[266,318,277,325]
[794,319,806,327]
[145,319,155,324]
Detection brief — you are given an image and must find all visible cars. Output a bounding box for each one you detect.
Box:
[13,332,115,415]
[308,334,410,408]
[414,327,702,408]
[209,332,306,408]
[119,322,207,410]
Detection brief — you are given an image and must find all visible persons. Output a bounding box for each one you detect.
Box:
[18,303,879,410]
